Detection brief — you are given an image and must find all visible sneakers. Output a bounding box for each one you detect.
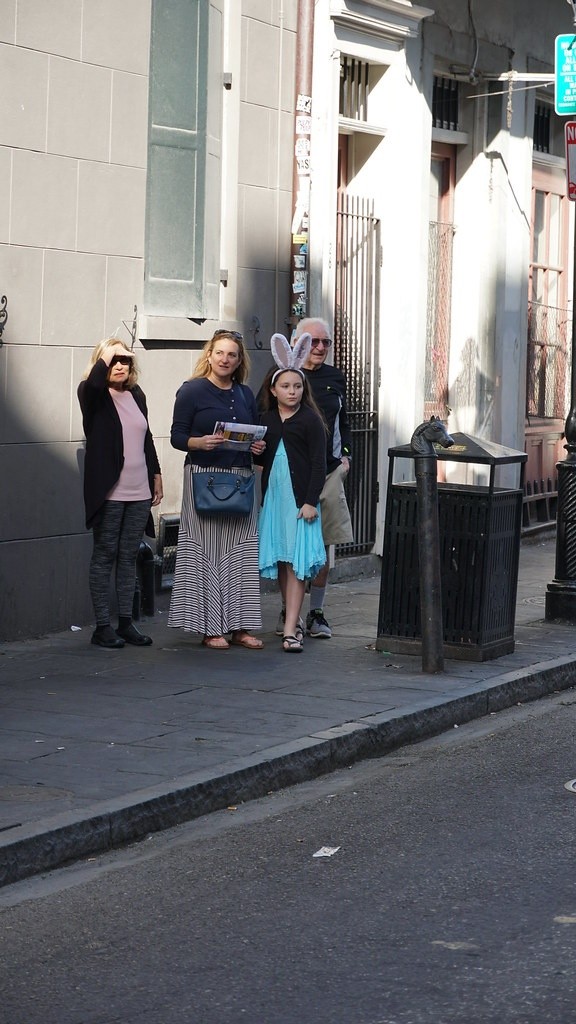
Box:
[276,609,286,635]
[306,608,332,638]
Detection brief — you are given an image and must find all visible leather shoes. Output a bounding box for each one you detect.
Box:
[115,623,153,646]
[91,626,125,648]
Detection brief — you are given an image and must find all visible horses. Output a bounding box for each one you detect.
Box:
[411,415,455,456]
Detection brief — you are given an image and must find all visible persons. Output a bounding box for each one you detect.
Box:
[258,367,328,653]
[171,328,268,650]
[256,317,356,638]
[78,338,166,649]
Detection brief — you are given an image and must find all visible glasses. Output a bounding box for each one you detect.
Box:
[212,328,243,341]
[312,337,332,347]
[109,356,131,366]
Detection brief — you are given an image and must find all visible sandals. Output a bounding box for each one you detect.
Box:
[202,635,230,649]
[281,636,304,652]
[228,636,264,648]
[295,624,307,644]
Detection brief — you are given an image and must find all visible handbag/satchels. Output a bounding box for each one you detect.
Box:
[192,472,256,517]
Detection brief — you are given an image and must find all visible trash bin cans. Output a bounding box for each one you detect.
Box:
[374,430,532,662]
